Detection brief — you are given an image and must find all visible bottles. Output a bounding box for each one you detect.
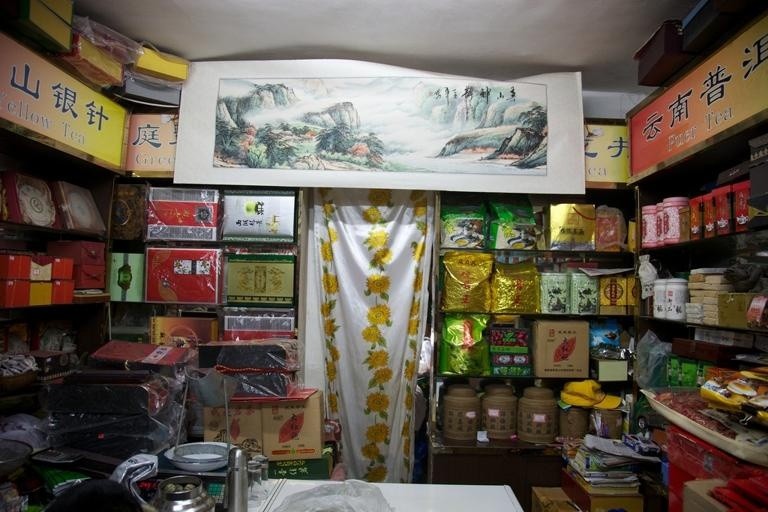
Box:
[150,475,215,511]
[642,198,688,246]
[45,422,63,455]
[227,448,248,511]
[443,385,589,444]
[652,277,688,320]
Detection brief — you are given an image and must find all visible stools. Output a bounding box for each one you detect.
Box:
[560,467,644,512]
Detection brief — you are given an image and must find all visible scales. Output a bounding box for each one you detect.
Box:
[155,442,247,512]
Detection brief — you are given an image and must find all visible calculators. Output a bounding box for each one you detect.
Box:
[26,447,85,468]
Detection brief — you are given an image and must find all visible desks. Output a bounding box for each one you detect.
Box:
[232,473,525,511]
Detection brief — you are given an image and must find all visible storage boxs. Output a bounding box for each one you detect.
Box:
[265,437,338,481]
[141,181,223,251]
[216,185,300,249]
[200,392,277,460]
[2,235,107,309]
[0,0,192,115]
[217,246,300,308]
[260,387,326,463]
[140,239,225,306]
[663,424,768,512]
[528,319,591,384]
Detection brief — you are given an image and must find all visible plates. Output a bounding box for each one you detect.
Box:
[164,439,240,471]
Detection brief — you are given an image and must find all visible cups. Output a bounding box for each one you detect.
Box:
[249,460,264,507]
[252,455,269,497]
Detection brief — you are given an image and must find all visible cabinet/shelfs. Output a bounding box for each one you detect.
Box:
[623,105,768,437]
[0,123,111,445]
[428,192,638,511]
[111,179,303,400]
